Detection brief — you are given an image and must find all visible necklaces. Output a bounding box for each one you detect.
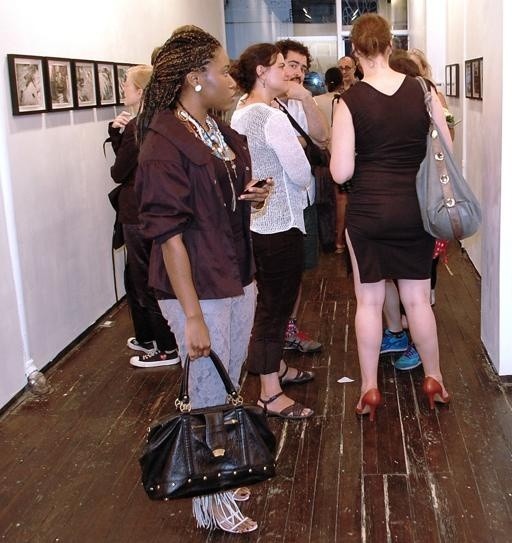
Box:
[174,100,238,213]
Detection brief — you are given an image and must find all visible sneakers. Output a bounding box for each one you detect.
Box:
[380,329,422,370]
[128,337,180,367]
[283,327,322,352]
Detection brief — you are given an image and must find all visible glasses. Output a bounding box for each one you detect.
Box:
[337,66,354,71]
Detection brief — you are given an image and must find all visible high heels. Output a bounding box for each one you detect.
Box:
[423,377,449,409]
[192,486,258,533]
[355,389,380,421]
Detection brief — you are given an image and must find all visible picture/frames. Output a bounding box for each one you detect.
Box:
[445,63,459,98]
[7,54,147,116]
[465,57,483,101]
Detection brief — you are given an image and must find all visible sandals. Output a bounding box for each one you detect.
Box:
[256,392,313,418]
[276,367,313,383]
[335,243,345,253]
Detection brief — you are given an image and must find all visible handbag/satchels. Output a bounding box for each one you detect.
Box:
[415,119,480,239]
[304,145,333,205]
[139,348,276,500]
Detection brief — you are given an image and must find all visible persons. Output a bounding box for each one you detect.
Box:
[228,42,316,419]
[328,12,482,422]
[135,25,276,534]
[103,64,181,369]
[209,40,457,371]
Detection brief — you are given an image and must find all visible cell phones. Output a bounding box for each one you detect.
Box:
[239,178,273,198]
